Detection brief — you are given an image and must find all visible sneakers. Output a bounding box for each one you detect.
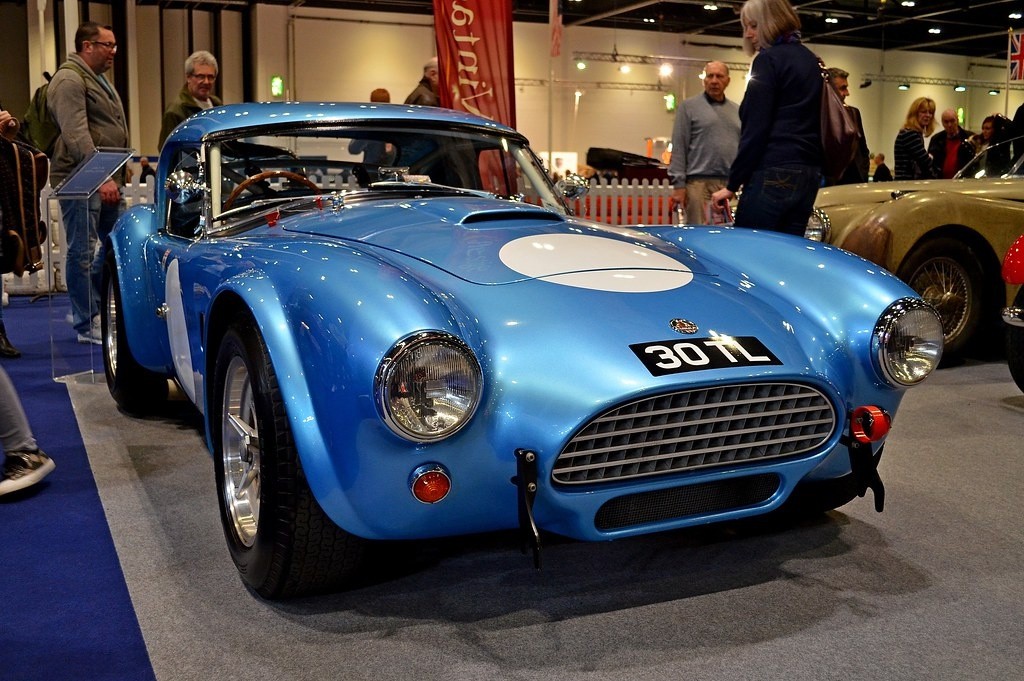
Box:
[0,446,56,495]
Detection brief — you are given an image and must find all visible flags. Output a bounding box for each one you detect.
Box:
[1009,32,1024,81]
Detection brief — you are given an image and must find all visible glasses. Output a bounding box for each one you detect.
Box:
[91,41,117,51]
[191,73,215,80]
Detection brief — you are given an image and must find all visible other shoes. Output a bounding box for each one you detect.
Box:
[77,315,102,344]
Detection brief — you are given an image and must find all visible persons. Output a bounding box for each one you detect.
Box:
[158,50,223,154]
[0,364,56,494]
[872,154,893,182]
[396,56,442,168]
[553,169,615,185]
[139,156,155,183]
[712,0,823,237]
[961,102,1024,176]
[45,21,130,345]
[820,66,871,186]
[666,59,741,227]
[348,88,395,166]
[894,97,936,180]
[929,107,977,179]
[0,106,22,359]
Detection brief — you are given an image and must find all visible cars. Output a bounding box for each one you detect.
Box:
[101,101,947,600]
[804,137,1024,364]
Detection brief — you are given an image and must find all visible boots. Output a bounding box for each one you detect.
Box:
[0,319,20,357]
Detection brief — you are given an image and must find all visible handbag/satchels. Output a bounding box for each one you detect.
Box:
[817,56,861,186]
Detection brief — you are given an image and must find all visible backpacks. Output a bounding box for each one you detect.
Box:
[24,64,96,160]
[0,115,50,276]
